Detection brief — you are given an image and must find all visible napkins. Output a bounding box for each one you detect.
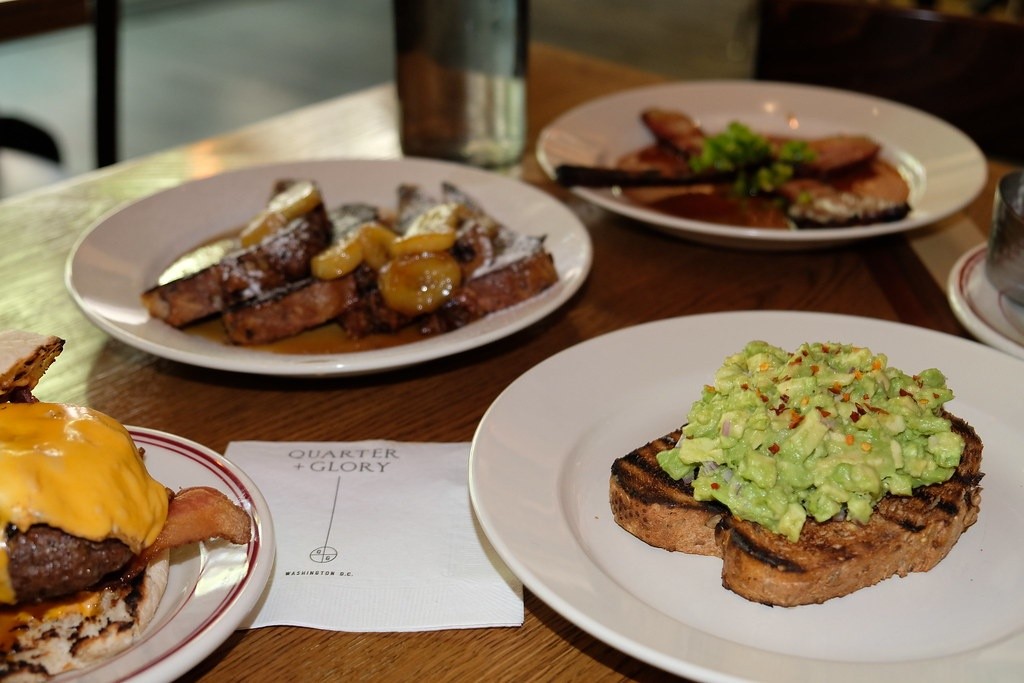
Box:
[221,439,525,633]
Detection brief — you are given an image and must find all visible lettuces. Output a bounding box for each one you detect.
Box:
[690,121,816,198]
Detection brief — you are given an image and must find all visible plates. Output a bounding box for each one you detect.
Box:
[468,310,1024,682]
[64,152,595,375]
[534,83,986,249]
[0,423,276,681]
[948,239,1024,361]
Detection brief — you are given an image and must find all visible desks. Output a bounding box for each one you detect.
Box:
[1,39,1023,683]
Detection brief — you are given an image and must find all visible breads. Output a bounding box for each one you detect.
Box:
[609,403,987,607]
[143,178,560,343]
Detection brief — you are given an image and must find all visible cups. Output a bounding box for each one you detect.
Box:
[982,172,1024,305]
[390,0,528,172]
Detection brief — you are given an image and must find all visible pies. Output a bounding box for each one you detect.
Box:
[0,329,66,401]
[0,548,171,683]
